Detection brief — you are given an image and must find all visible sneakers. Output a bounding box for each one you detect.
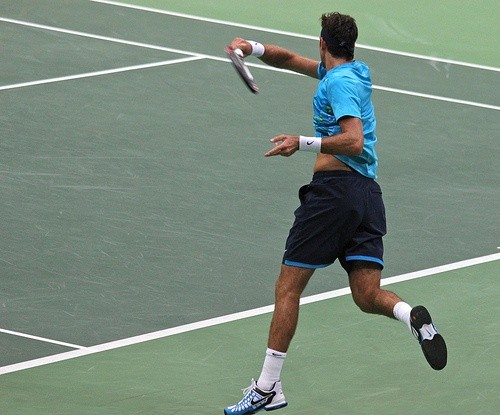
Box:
[224,378,288,415]
[410,305,448,371]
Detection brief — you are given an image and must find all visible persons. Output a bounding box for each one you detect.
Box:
[225,11,448,415]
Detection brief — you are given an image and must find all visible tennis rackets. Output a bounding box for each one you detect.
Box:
[225,46,260,95]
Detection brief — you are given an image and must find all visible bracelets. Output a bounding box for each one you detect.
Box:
[247,40,265,58]
[299,136,321,153]
